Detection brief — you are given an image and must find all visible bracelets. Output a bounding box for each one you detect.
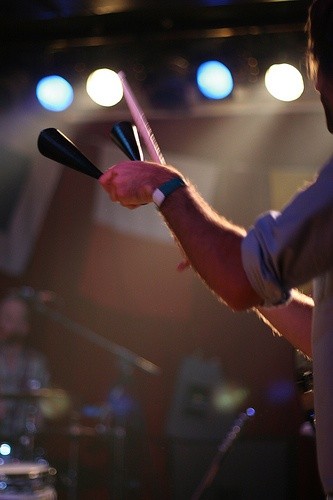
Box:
[151,176,186,208]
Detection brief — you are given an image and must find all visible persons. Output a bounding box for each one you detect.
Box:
[96,1,332,500]
